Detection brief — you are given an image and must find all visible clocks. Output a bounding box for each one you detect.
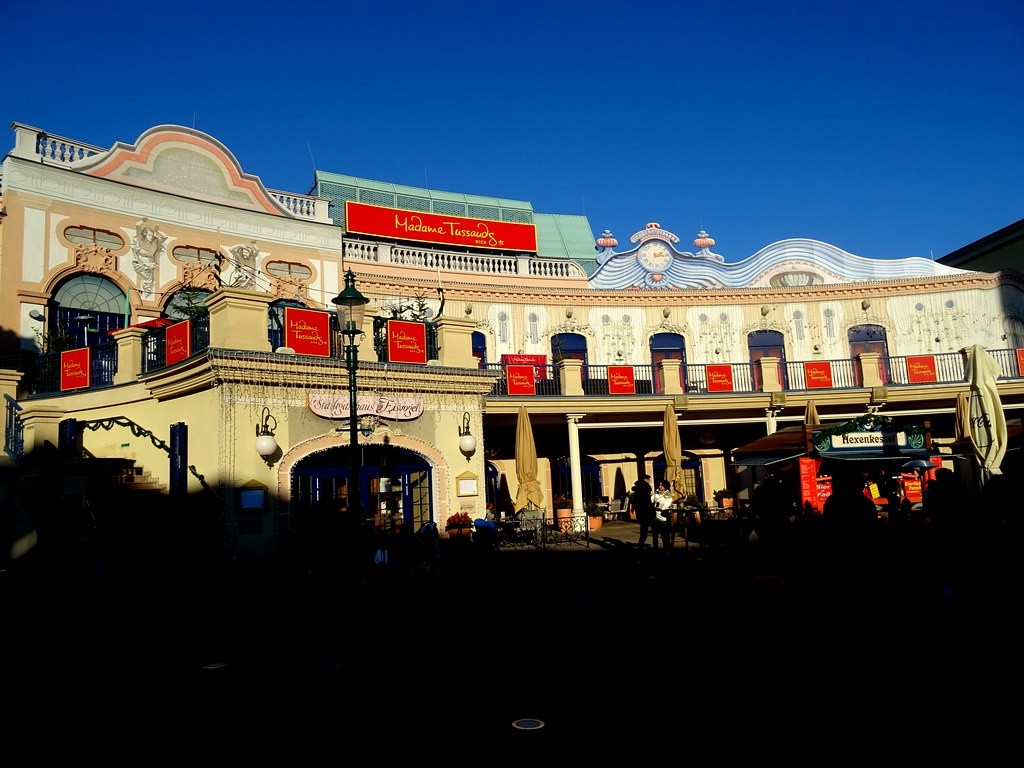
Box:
[638,239,674,272]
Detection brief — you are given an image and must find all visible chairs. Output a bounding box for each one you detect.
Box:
[611,498,629,522]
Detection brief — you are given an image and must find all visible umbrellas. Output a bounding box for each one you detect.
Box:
[803,399,821,429]
[953,345,1008,486]
[660,403,688,510]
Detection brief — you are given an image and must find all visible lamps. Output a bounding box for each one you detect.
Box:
[256,407,277,463]
[459,412,476,455]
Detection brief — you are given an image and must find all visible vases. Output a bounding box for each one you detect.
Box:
[557,509,572,532]
[449,529,471,543]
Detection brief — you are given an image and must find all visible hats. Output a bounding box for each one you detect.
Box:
[639,473,651,479]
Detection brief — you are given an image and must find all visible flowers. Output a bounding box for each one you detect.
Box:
[484,502,494,521]
[555,496,573,509]
[446,512,474,531]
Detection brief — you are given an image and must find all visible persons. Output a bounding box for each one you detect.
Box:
[806,465,1014,562]
[632,473,660,549]
[650,479,676,550]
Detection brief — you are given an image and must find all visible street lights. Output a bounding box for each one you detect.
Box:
[334,269,369,525]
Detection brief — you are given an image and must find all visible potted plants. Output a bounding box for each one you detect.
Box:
[713,489,734,508]
[585,502,605,530]
